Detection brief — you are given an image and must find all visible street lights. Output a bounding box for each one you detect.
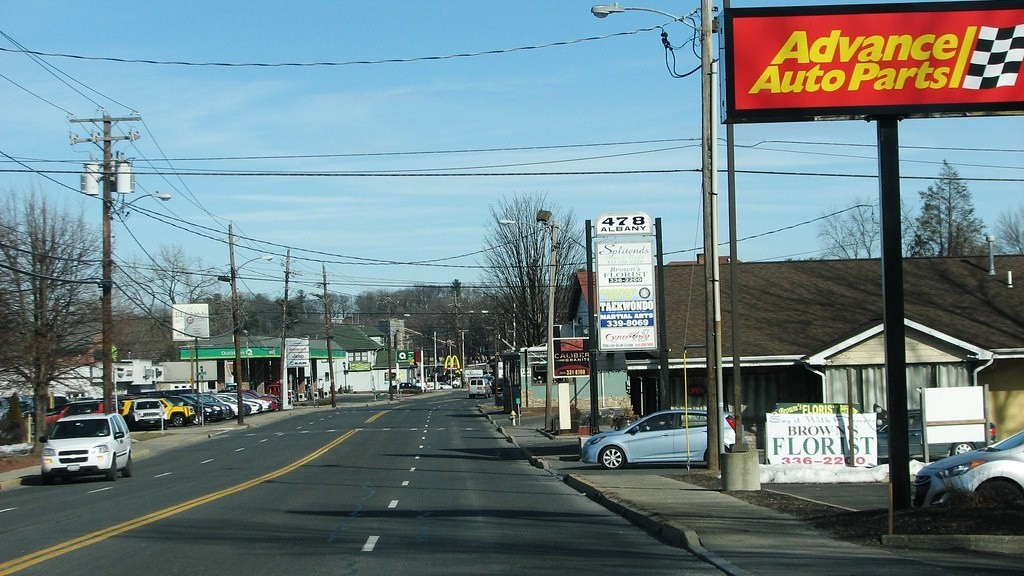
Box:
[590,5,719,478]
[101,193,172,412]
[217,255,272,425]
[498,220,558,431]
[387,314,410,400]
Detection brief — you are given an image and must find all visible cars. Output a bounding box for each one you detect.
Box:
[168,390,278,426]
[438,382,453,390]
[877,408,995,457]
[389,382,426,394]
[484,376,496,386]
[912,428,1024,507]
[581,409,746,470]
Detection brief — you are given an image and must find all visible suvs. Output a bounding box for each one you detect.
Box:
[129,399,168,430]
[46,399,120,435]
[121,399,195,428]
[25,396,69,421]
[39,411,132,481]
[0,397,30,420]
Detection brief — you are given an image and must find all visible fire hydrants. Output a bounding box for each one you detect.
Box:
[508,410,516,426]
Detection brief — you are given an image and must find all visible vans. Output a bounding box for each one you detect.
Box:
[469,378,492,399]
